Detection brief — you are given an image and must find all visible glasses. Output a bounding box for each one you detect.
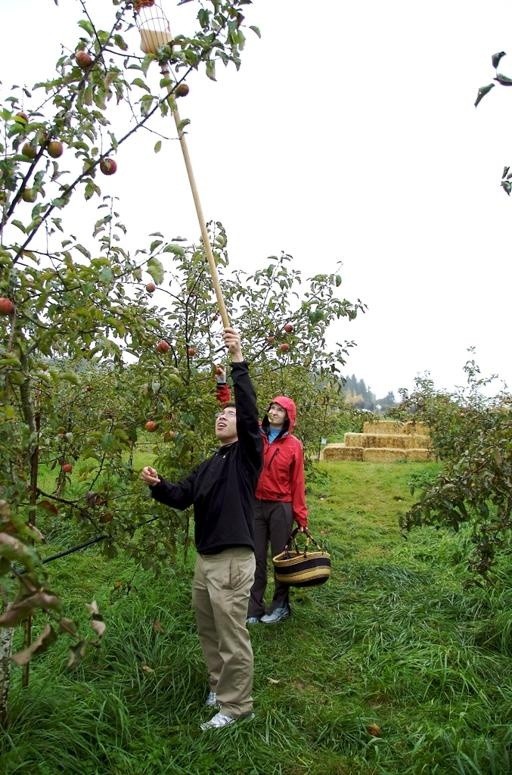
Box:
[215,412,235,418]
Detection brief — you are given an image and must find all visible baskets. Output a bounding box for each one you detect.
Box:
[272,527,331,587]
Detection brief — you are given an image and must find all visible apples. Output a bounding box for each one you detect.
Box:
[0,0,294,472]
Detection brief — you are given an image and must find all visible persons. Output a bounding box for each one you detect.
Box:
[215,365,308,624]
[141,328,264,733]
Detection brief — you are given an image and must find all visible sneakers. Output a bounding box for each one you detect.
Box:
[198,691,255,731]
[246,605,290,624]
